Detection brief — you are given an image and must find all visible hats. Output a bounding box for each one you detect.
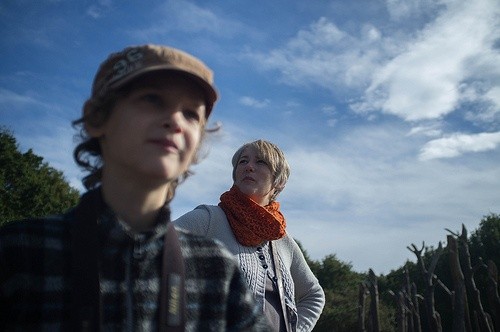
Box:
[91,44,219,120]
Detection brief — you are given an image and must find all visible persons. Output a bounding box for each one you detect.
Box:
[1,42,275,332]
[172,139,327,332]
